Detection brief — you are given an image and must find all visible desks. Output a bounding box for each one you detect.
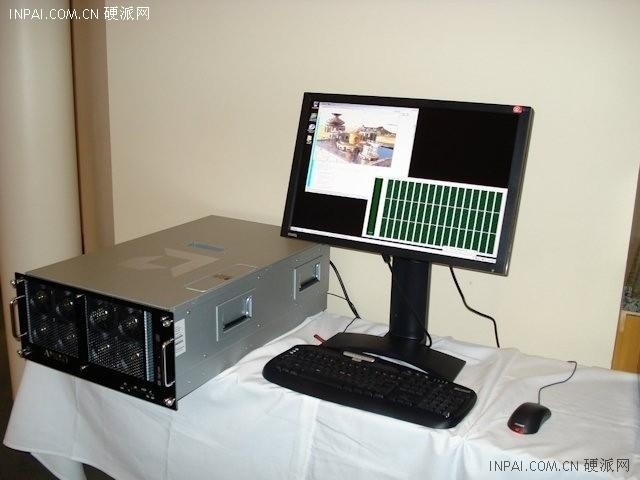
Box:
[24,311,640,480]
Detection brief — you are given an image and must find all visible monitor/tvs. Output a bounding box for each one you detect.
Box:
[279,91,534,383]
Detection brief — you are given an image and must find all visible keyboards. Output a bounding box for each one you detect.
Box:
[263,344,478,430]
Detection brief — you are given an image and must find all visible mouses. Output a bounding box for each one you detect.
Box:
[507,402,551,434]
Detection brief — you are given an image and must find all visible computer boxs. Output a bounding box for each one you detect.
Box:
[10,215,331,411]
